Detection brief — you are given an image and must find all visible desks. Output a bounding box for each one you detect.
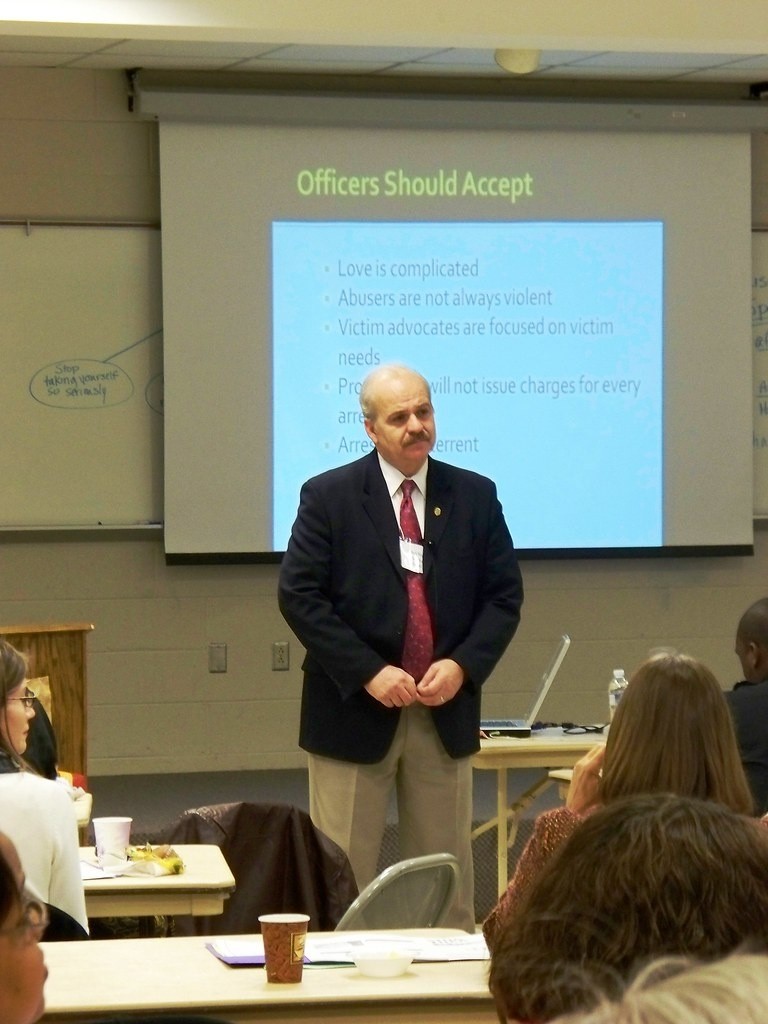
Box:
[77,844,236,938]
[468,725,612,900]
[34,925,501,1024]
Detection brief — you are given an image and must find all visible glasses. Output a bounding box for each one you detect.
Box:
[0,891,48,941]
[563,725,603,734]
[4,689,36,707]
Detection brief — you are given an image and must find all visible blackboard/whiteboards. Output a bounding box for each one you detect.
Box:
[0,215,165,532]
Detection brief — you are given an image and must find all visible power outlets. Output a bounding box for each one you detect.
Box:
[272,641,289,670]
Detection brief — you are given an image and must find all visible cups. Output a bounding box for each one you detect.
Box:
[92,816,133,871]
[348,945,414,978]
[258,913,310,985]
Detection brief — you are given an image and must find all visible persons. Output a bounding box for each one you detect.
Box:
[0,830,49,1024]
[719,597,768,817]
[487,793,768,1024]
[481,652,758,960]
[275,368,523,938]
[0,641,90,938]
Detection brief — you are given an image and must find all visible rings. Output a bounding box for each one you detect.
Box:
[440,695,446,703]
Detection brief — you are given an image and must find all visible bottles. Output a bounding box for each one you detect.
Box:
[608,669,631,725]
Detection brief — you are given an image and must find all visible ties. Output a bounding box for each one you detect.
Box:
[400,479,435,684]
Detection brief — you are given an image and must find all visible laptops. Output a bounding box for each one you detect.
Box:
[479,634,571,738]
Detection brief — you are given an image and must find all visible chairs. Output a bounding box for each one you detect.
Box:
[333,853,459,931]
